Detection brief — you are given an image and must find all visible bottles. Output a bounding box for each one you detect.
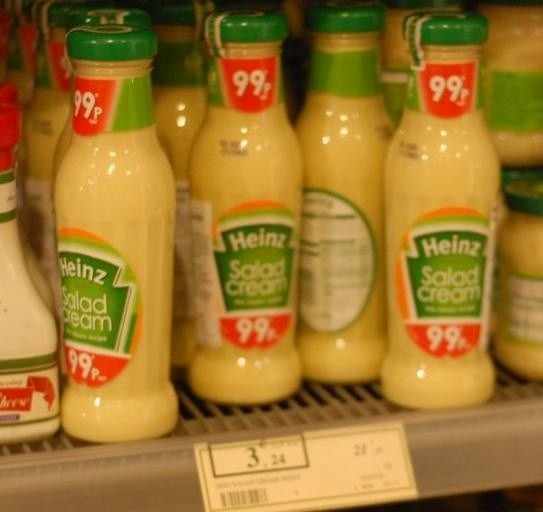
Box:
[0,105,59,444]
[153,0,222,368]
[23,0,92,256]
[294,6,398,382]
[52,7,153,208]
[188,11,303,404]
[493,179,543,382]
[3,2,38,105]
[477,0,542,169]
[52,23,180,442]
[0,82,17,104]
[380,12,503,410]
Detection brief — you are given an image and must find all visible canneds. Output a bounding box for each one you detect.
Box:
[385,0,543,381]
